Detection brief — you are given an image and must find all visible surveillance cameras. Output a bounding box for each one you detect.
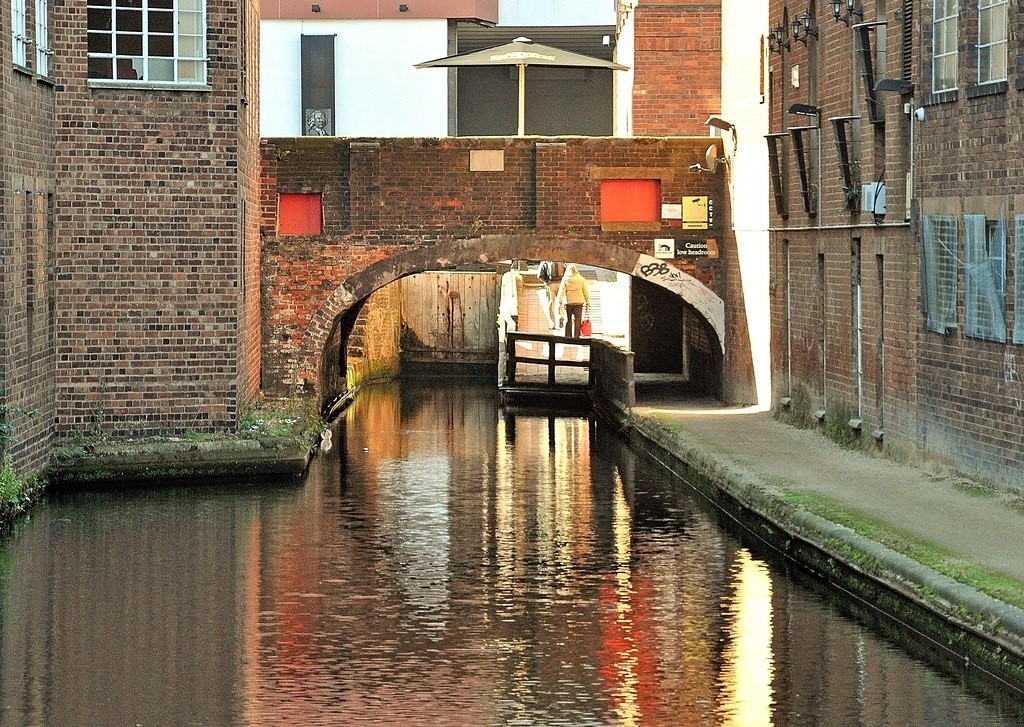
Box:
[915,107,924,121]
[603,35,611,47]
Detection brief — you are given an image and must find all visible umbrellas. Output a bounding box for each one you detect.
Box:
[411,37,631,135]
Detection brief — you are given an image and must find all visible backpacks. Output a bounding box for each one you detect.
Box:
[547,261,566,281]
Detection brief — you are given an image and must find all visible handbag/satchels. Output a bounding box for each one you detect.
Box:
[581,305,591,336]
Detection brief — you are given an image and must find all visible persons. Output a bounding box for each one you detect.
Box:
[537,261,567,329]
[555,265,591,338]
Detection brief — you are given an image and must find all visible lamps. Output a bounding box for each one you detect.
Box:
[872,78,913,96]
[689,117,737,175]
[767,21,791,55]
[829,0,864,28]
[785,104,819,118]
[601,33,615,47]
[791,8,819,47]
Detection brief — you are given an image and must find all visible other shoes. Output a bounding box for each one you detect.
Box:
[559,317,564,328]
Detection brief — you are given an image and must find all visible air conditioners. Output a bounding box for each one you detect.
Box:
[862,181,886,215]
